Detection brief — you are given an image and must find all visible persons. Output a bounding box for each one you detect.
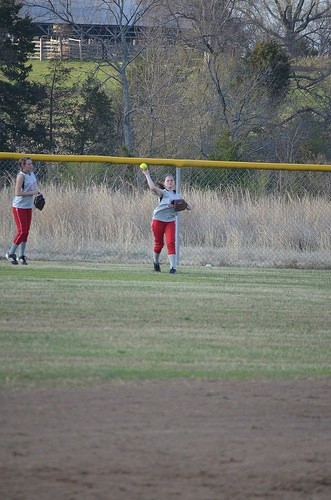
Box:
[5,157,39,265]
[141,163,191,274]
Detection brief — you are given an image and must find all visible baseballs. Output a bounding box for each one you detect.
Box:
[140,163,147,170]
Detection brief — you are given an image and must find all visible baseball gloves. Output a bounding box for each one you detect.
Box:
[171,199,187,212]
[34,192,45,211]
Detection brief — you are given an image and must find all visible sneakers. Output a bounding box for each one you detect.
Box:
[154,261,161,273]
[169,267,177,274]
[5,251,29,267]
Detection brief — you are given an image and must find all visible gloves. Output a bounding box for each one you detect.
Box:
[33,194,46,211]
